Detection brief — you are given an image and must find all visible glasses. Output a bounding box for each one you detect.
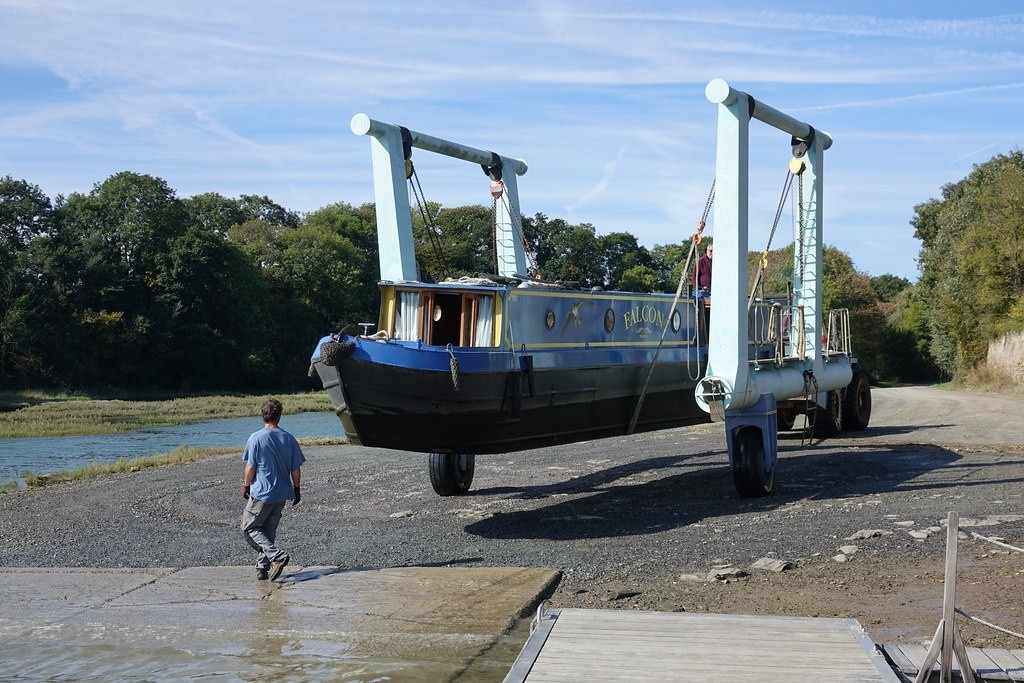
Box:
[707,248,712,252]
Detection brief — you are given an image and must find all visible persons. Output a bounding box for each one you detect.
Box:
[690,243,712,299]
[241,399,307,581]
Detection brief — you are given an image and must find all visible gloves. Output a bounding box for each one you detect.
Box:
[291,486,301,506]
[240,485,250,500]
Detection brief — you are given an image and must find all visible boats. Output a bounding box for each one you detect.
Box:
[309,279,799,456]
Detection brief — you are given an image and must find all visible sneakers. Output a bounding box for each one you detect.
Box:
[269,556,289,580]
[257,569,268,580]
